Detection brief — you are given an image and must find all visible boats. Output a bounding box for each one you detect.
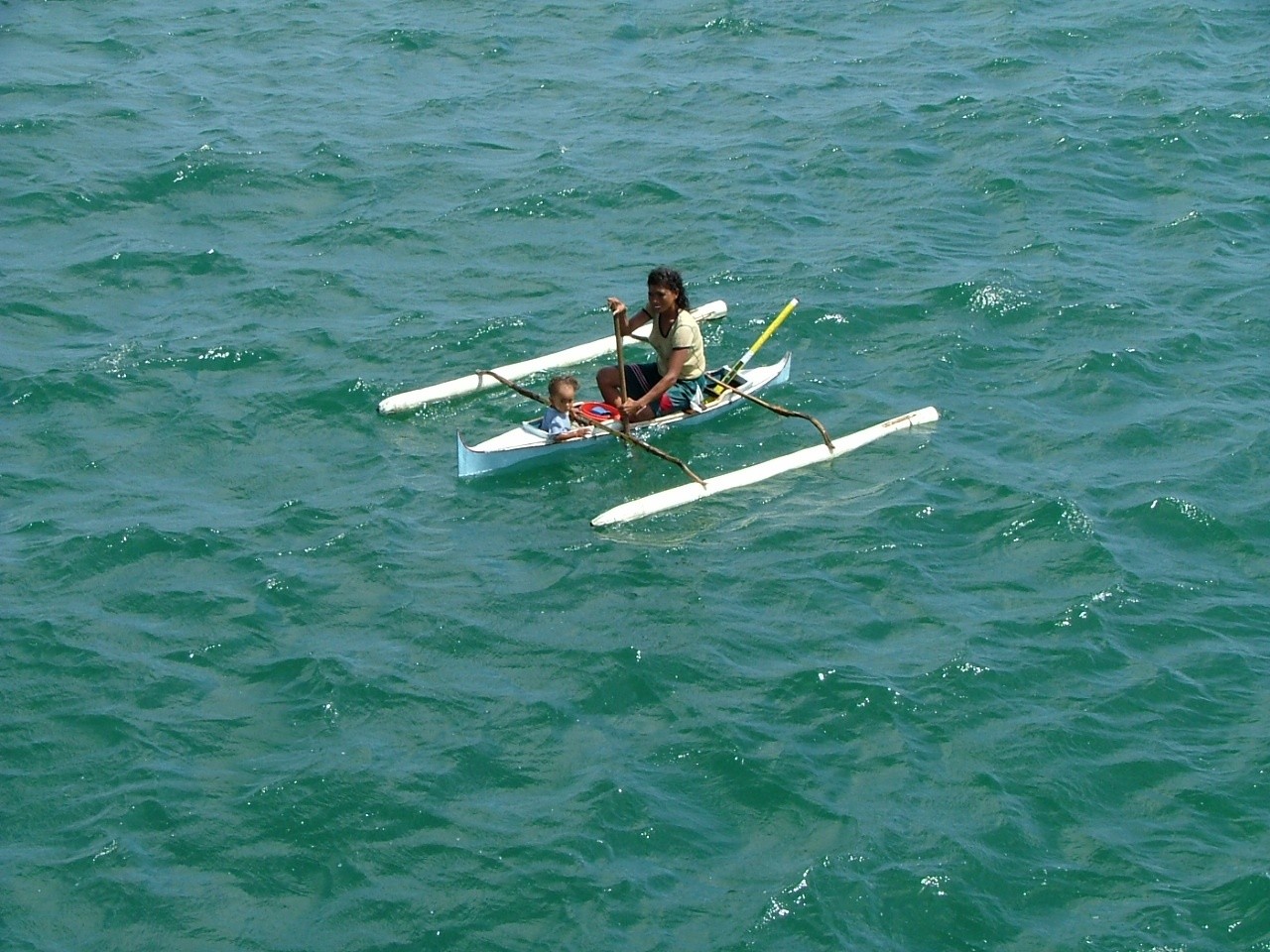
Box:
[374,299,939,528]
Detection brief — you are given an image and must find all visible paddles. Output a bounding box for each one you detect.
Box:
[609,303,631,445]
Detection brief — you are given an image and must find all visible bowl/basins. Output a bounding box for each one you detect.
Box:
[578,402,621,421]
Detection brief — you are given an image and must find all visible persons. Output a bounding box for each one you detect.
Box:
[596,267,708,424]
[539,374,592,443]
